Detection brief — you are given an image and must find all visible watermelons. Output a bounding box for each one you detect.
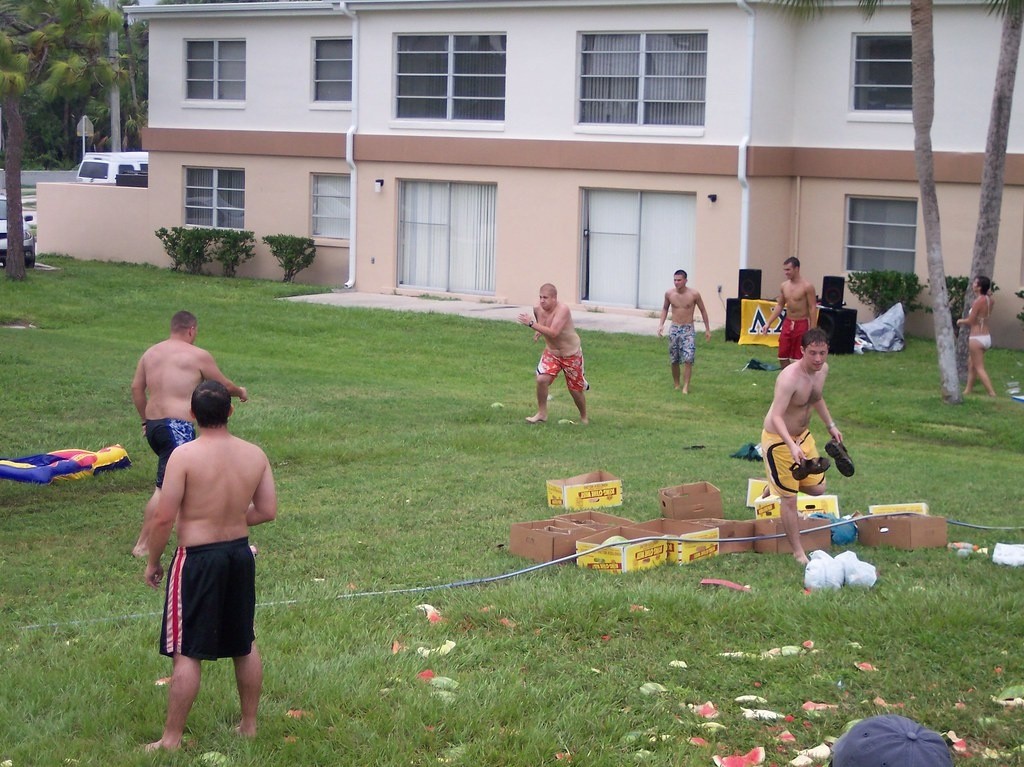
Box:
[599,535,631,549]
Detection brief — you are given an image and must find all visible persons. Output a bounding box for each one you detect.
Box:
[518,283,590,426]
[762,257,817,370]
[145,380,276,751]
[957,276,996,396]
[131,310,248,558]
[657,270,711,394]
[761,327,842,565]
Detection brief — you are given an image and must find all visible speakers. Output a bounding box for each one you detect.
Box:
[817,307,858,353]
[821,275,845,307]
[738,268,762,299]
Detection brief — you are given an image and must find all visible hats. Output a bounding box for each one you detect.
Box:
[830,714,951,767]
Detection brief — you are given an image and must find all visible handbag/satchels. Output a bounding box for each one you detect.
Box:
[834,550,876,588]
[804,550,844,590]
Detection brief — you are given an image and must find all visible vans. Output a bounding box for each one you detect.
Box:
[76,151,148,184]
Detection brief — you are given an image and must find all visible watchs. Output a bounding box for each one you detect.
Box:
[529,321,533,327]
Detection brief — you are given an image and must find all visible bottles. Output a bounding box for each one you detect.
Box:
[948,543,978,552]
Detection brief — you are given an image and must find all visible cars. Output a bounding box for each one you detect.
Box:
[0,193,36,268]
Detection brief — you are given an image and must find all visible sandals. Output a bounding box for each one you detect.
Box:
[825,436,854,477]
[789,457,830,480]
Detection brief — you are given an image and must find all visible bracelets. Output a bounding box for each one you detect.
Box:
[826,422,835,429]
[142,422,147,427]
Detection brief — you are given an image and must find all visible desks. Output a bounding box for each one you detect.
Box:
[725,298,857,357]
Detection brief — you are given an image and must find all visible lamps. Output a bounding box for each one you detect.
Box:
[376,179,384,193]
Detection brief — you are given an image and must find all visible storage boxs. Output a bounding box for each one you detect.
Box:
[856,515,948,548]
[554,510,633,532]
[753,517,832,554]
[869,503,930,516]
[659,480,724,520]
[754,493,841,519]
[689,517,755,554]
[575,526,667,576]
[632,518,720,566]
[510,519,592,564]
[746,477,770,508]
[545,470,623,509]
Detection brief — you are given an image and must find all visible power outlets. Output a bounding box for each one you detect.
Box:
[717,284,723,293]
